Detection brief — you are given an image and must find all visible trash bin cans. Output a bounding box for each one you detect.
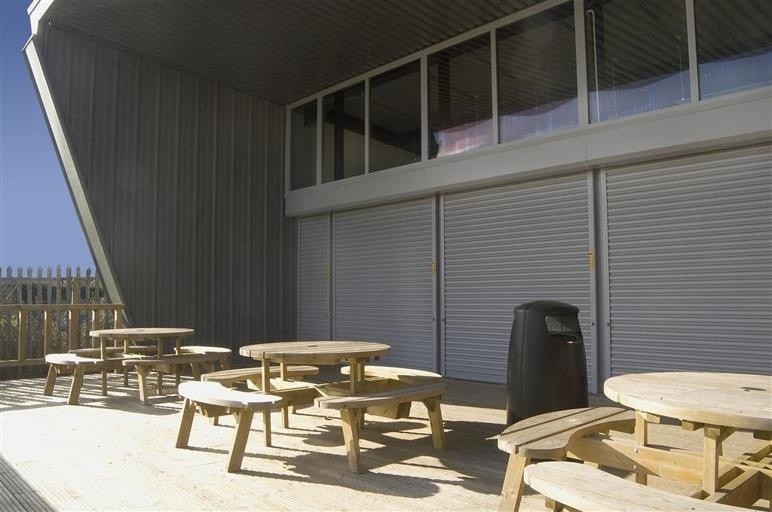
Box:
[505,300,587,464]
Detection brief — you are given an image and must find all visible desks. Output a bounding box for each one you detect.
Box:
[239,340,391,447]
[89,327,195,396]
[602,371,772,511]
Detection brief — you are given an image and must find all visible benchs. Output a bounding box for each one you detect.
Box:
[44,345,233,407]
[178,365,448,473]
[497,406,766,512]
